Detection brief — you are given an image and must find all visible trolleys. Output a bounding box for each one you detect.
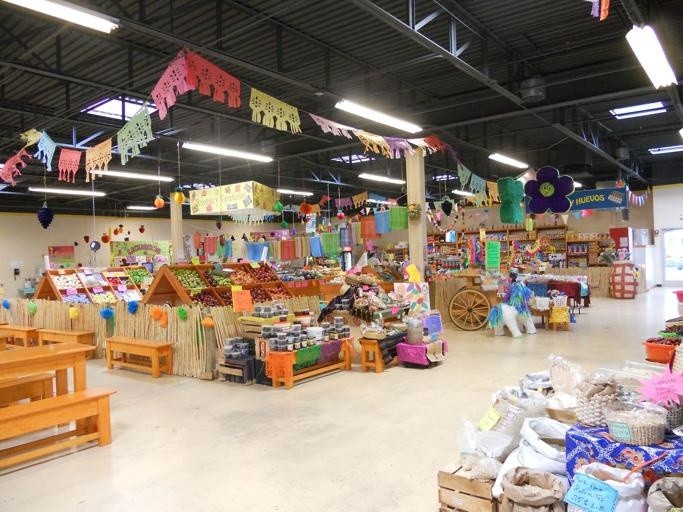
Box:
[446,268,558,331]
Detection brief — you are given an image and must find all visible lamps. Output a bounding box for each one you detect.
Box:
[488,152,529,171]
[0,0,122,35]
[335,99,423,135]
[626,22,678,91]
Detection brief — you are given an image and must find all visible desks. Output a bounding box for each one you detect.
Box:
[396,339,448,368]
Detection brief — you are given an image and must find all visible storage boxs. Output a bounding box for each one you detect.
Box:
[423,229,632,273]
[40,264,156,305]
[565,290,683,489]
[146,263,297,310]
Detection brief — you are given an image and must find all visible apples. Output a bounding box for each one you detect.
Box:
[250,267,276,282]
[171,269,232,288]
[190,290,232,306]
[244,286,272,303]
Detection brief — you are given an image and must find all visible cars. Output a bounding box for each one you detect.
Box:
[665,248,683,272]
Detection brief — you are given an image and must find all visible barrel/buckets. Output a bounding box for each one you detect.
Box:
[608,259,638,299]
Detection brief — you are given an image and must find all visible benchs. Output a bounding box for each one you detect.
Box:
[0,322,173,475]
[271,335,405,389]
